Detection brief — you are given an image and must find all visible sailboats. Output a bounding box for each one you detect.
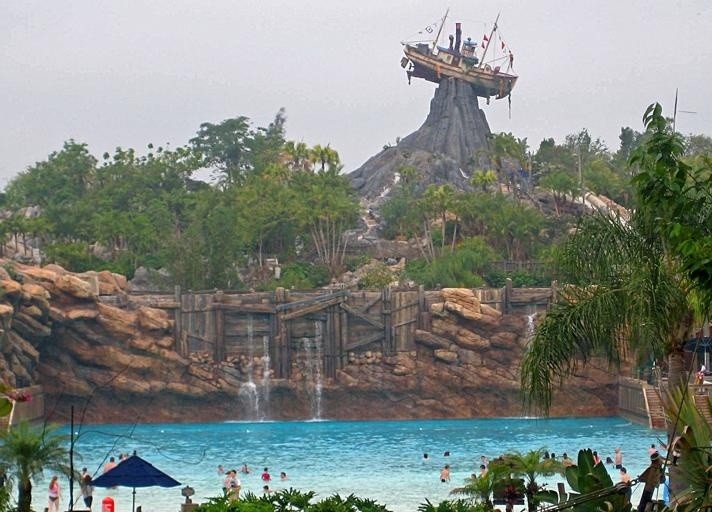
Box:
[399,7,519,99]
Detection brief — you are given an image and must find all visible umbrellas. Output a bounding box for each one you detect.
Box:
[89,450,182,512]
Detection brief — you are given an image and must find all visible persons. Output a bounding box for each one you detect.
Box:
[440,466,450,482]
[82,475,93,511]
[263,485,277,493]
[104,453,129,490]
[470,447,630,485]
[261,467,271,481]
[648,443,657,455]
[700,361,707,373]
[216,462,251,500]
[281,471,287,480]
[81,468,89,479]
[693,368,705,393]
[47,476,62,512]
